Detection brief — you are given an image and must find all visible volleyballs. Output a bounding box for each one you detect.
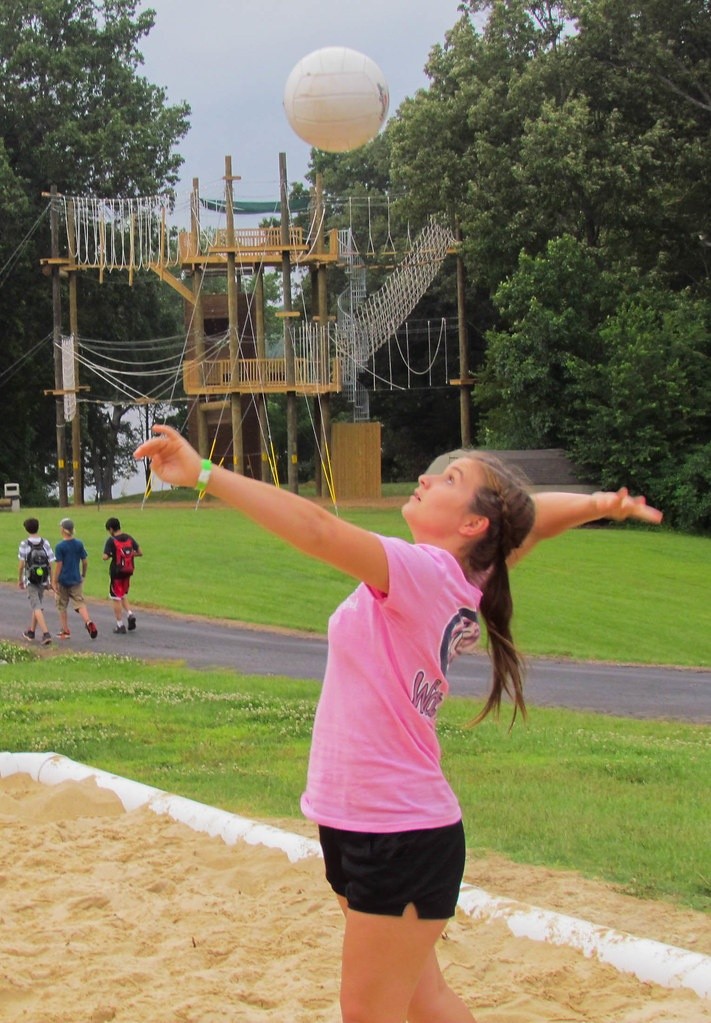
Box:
[282,47,392,156]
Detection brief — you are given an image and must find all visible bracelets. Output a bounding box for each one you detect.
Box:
[195,459,212,490]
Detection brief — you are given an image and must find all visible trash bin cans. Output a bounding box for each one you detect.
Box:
[4,482,20,509]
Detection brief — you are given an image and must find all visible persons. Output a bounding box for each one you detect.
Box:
[17,518,56,645]
[104,518,142,633]
[51,519,98,640]
[133,424,662,1022]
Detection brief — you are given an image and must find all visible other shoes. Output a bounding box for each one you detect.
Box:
[86,620,98,639]
[127,614,137,630]
[113,625,126,634]
[41,634,52,646]
[21,630,35,640]
[55,630,72,639]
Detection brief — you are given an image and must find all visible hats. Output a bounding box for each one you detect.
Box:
[60,518,76,536]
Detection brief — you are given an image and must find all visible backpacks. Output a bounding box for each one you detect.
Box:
[26,537,50,584]
[109,533,134,576]
